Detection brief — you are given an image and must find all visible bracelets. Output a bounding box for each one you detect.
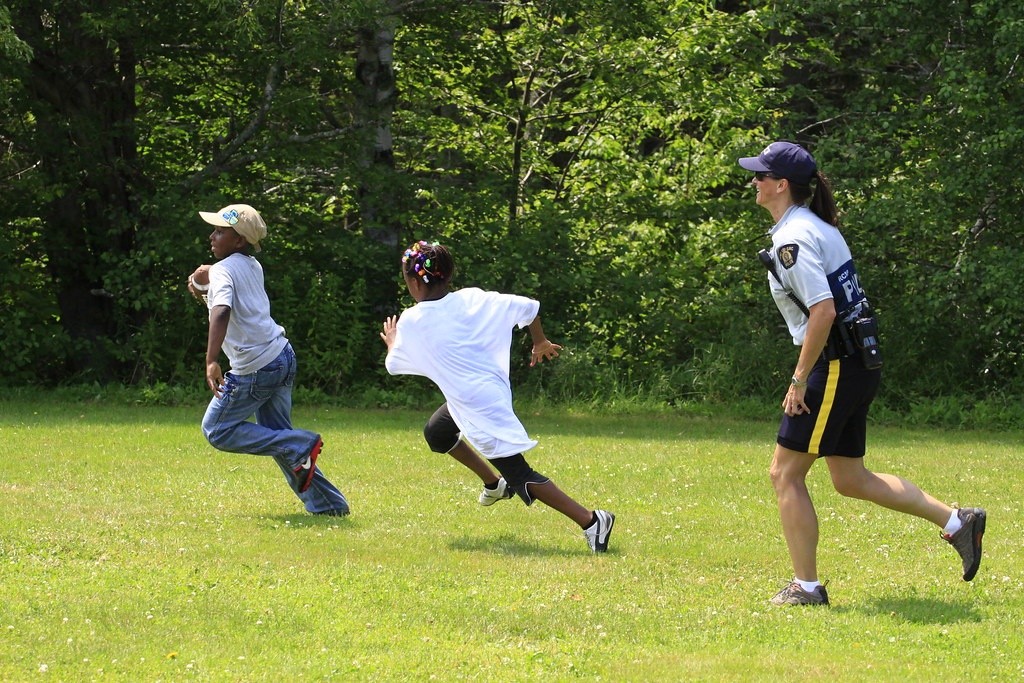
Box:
[531,341,552,354]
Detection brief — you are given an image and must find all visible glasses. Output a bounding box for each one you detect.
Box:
[754,171,779,183]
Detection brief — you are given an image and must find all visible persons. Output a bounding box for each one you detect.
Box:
[750,142,987,606]
[186,205,350,517]
[379,240,615,553]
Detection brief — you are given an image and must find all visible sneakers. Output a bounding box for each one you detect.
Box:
[945,507,988,580]
[296,435,323,493]
[477,476,515,507]
[771,583,829,607]
[585,509,615,554]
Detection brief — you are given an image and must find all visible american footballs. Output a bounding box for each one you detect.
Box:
[191,265,212,305]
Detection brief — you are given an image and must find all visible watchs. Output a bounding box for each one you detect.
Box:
[791,376,808,387]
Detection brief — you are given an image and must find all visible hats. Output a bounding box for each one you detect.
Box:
[199,204,267,246]
[738,142,819,183]
